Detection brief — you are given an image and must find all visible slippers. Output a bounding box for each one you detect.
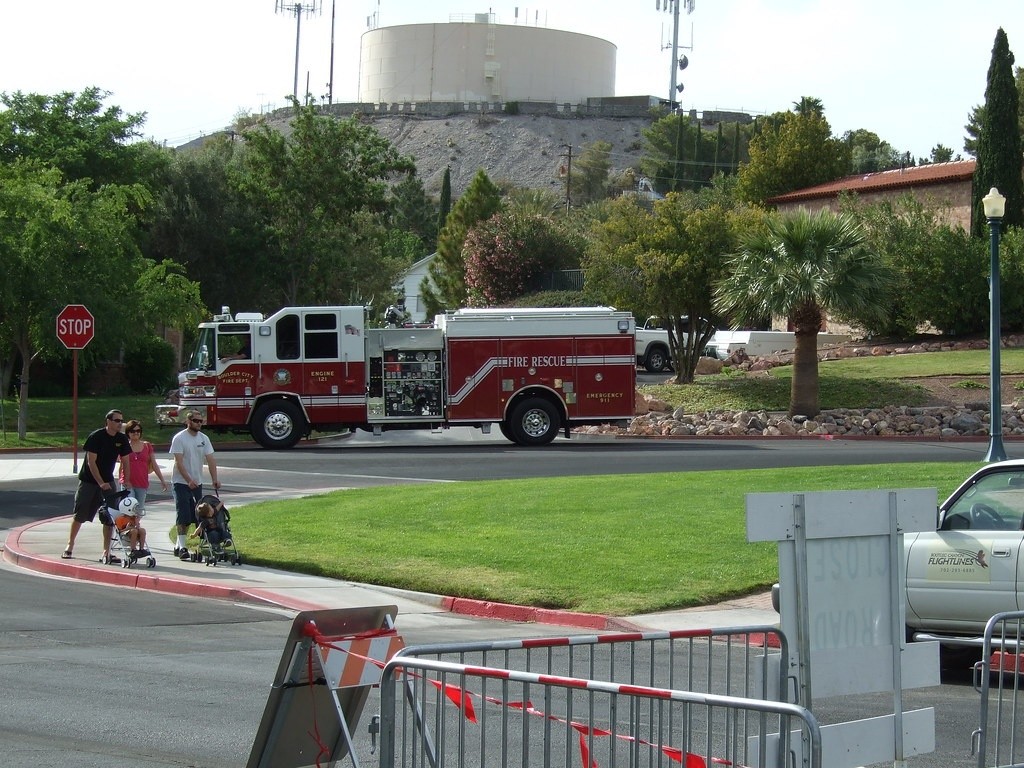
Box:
[99,556,122,563]
[62,551,72,559]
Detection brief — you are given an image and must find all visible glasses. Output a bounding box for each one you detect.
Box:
[110,418,124,423]
[127,429,141,433]
[189,418,203,423]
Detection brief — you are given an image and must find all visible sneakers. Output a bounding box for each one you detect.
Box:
[174,547,180,557]
[179,548,191,561]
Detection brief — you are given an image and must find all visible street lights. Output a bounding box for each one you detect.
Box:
[978,186,1010,462]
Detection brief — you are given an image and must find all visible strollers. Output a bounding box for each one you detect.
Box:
[187,483,242,566]
[101,482,157,569]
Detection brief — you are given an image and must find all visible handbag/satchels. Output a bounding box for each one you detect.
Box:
[144,442,154,474]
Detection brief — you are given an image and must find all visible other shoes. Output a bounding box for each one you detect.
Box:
[214,549,224,554]
[131,549,142,557]
[224,539,231,547]
[139,549,151,555]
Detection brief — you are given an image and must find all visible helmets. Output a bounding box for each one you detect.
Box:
[119,497,139,516]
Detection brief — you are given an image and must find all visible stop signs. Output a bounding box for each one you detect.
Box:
[56,303,95,350]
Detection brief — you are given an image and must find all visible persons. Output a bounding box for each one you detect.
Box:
[115,496,146,551]
[169,410,222,560]
[118,420,168,520]
[190,498,232,554]
[61,409,134,562]
[220,333,251,363]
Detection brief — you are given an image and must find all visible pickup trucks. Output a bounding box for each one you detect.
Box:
[632,314,722,372]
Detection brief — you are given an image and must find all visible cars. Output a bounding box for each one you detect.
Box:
[771,459,1024,678]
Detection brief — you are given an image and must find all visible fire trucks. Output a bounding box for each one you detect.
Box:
[155,299,639,449]
